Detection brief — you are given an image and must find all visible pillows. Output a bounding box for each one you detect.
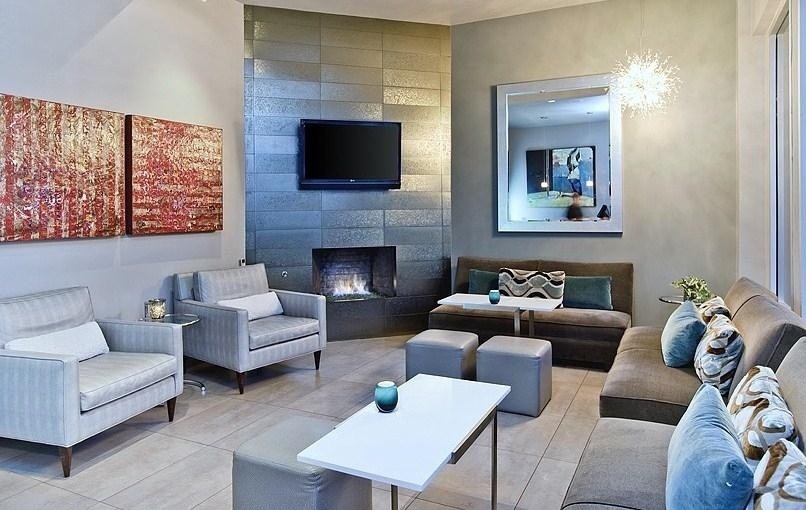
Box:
[2,316,110,371]
[741,440,806,510]
[213,288,282,325]
[464,265,498,295]
[564,274,612,309]
[686,315,744,398]
[648,384,755,507]
[496,266,565,309]
[696,295,732,322]
[724,364,799,460]
[659,301,707,369]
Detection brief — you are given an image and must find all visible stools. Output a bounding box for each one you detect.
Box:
[401,327,478,391]
[471,333,555,417]
[232,418,375,510]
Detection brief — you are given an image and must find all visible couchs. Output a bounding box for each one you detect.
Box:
[599,276,805,426]
[428,255,643,377]
[560,334,806,509]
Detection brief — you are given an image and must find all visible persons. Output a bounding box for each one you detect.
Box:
[567,192,583,220]
[554,148,583,199]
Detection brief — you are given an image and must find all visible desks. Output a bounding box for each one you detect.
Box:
[655,295,712,315]
[295,373,518,510]
[142,311,208,408]
[436,292,565,336]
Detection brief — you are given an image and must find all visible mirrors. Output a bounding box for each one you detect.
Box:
[496,72,621,239]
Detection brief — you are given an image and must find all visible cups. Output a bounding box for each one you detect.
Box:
[489,290,501,304]
[148,298,168,320]
[373,380,399,413]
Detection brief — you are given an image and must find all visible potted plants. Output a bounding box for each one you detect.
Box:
[671,276,709,302]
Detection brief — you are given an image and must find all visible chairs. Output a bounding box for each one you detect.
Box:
[170,262,326,398]
[0,285,184,480]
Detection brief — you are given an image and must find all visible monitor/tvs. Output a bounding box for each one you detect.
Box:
[297,118,402,191]
